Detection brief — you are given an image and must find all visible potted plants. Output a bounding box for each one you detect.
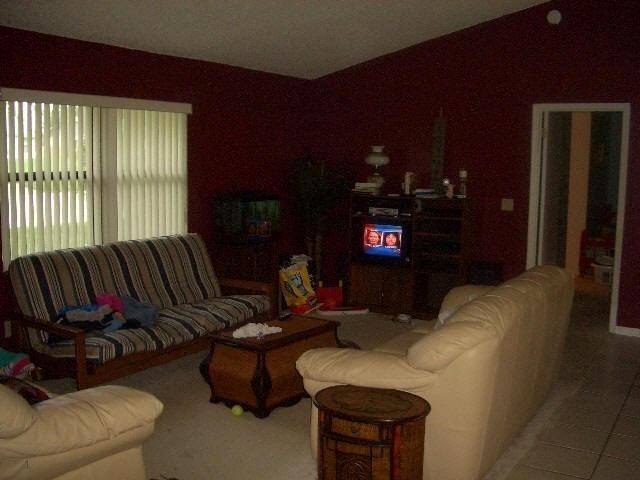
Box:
[296,160,349,309]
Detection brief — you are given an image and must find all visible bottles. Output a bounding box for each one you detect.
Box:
[366,144,390,180]
[405,171,416,195]
[459,167,468,196]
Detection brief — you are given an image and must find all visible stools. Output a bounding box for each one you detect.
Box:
[590,255,614,283]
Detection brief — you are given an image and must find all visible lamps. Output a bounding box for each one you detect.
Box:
[365,146,390,183]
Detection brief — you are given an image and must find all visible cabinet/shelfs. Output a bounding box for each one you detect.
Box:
[349,195,466,320]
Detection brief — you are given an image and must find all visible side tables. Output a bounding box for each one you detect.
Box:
[312,385,430,480]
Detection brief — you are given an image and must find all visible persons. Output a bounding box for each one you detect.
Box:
[366,231,381,247]
[384,232,399,248]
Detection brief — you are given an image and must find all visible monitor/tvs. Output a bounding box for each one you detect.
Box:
[363,223,402,256]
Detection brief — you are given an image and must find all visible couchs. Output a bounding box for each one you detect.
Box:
[296,264,574,480]
[0,381,164,480]
[0,232,275,391]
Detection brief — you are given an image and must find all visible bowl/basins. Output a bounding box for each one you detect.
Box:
[591,261,613,285]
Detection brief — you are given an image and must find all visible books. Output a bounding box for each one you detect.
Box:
[352,181,379,197]
